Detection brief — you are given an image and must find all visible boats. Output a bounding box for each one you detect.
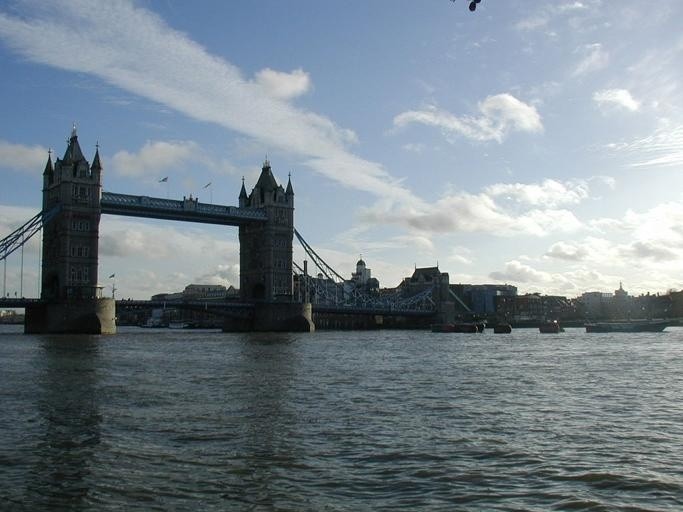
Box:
[583,318,671,334]
